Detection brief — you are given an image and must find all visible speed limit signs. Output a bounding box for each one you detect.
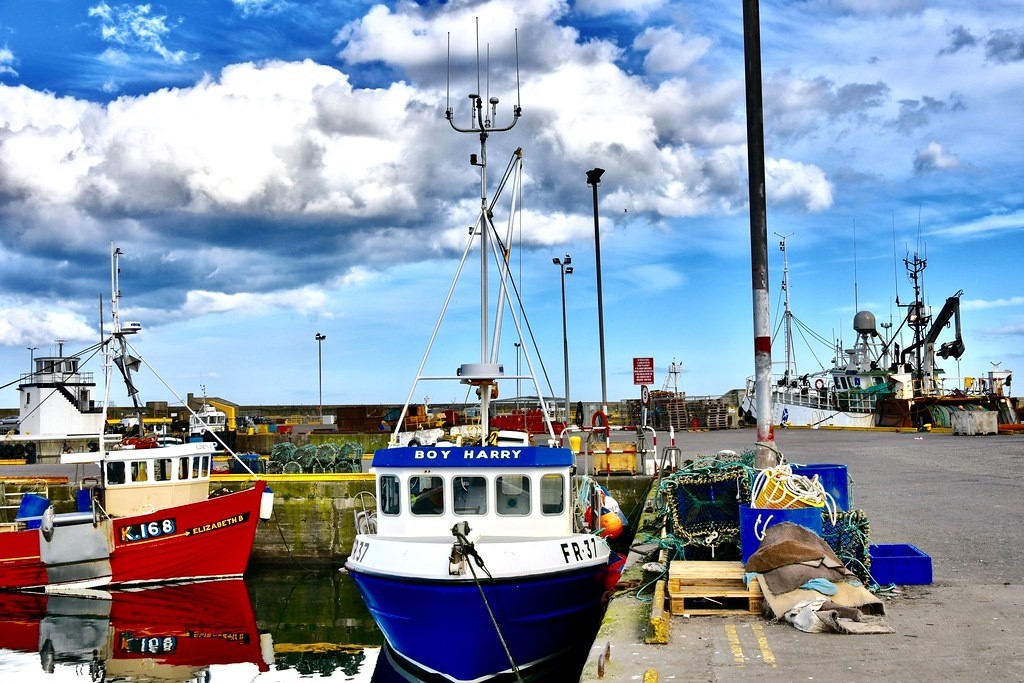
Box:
[641,386,650,406]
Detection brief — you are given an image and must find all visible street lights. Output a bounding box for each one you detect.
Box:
[315,332,326,421]
[552,255,575,425]
[585,167,611,416]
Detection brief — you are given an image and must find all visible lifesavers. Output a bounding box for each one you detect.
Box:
[815,378,825,390]
[591,410,608,434]
[121,436,159,449]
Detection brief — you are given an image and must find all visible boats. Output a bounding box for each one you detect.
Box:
[341,14,625,683]
[0,237,276,589]
[736,229,1024,429]
